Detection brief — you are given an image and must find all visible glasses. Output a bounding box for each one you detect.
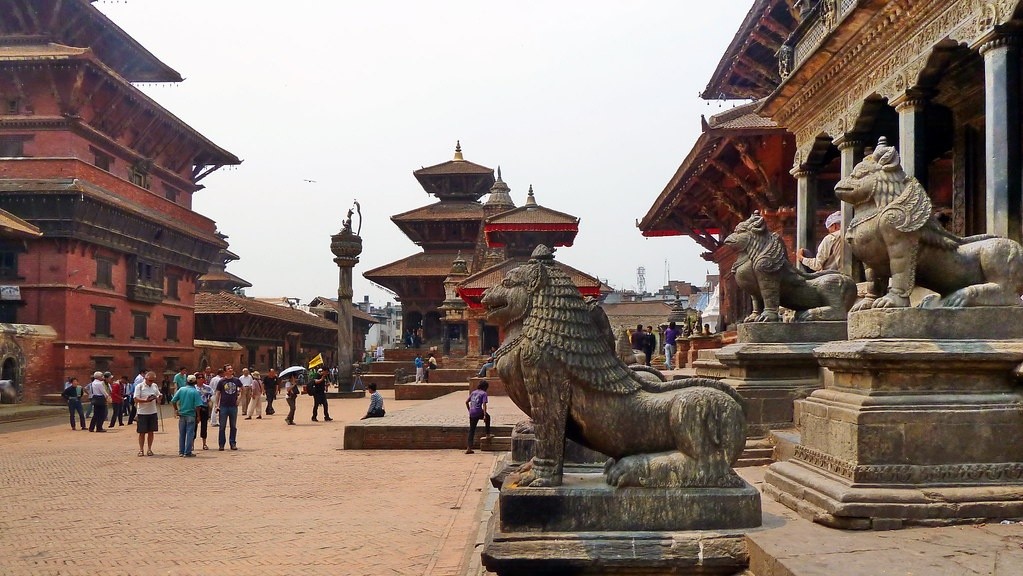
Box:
[198,377,204,379]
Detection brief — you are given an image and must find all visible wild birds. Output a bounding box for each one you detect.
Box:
[304,179,317,183]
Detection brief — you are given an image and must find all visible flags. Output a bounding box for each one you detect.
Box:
[308,354,322,368]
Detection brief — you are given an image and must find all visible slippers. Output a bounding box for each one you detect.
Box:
[486,434,494,439]
[465,451,474,454]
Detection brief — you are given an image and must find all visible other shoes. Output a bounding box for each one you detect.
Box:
[219,447,224,450]
[245,416,251,419]
[202,445,208,449]
[147,450,153,456]
[183,454,195,457]
[324,417,332,420]
[72,412,136,432]
[231,446,237,450]
[137,451,144,456]
[285,419,295,425]
[311,417,318,421]
[178,453,183,457]
[256,416,261,419]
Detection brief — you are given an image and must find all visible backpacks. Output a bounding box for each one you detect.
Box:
[306,380,314,396]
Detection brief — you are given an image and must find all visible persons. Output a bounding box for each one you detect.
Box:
[353,363,367,391]
[62,360,342,458]
[663,322,683,370]
[403,325,426,350]
[644,325,656,366]
[797,211,842,272]
[702,324,713,336]
[360,382,385,419]
[366,353,373,370]
[465,380,495,454]
[475,345,500,377]
[631,324,646,353]
[414,352,438,384]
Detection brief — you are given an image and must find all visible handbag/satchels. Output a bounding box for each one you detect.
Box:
[91,395,104,406]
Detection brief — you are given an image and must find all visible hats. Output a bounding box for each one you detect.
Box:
[825,211,842,228]
[101,372,113,379]
[251,371,261,379]
[187,375,195,382]
